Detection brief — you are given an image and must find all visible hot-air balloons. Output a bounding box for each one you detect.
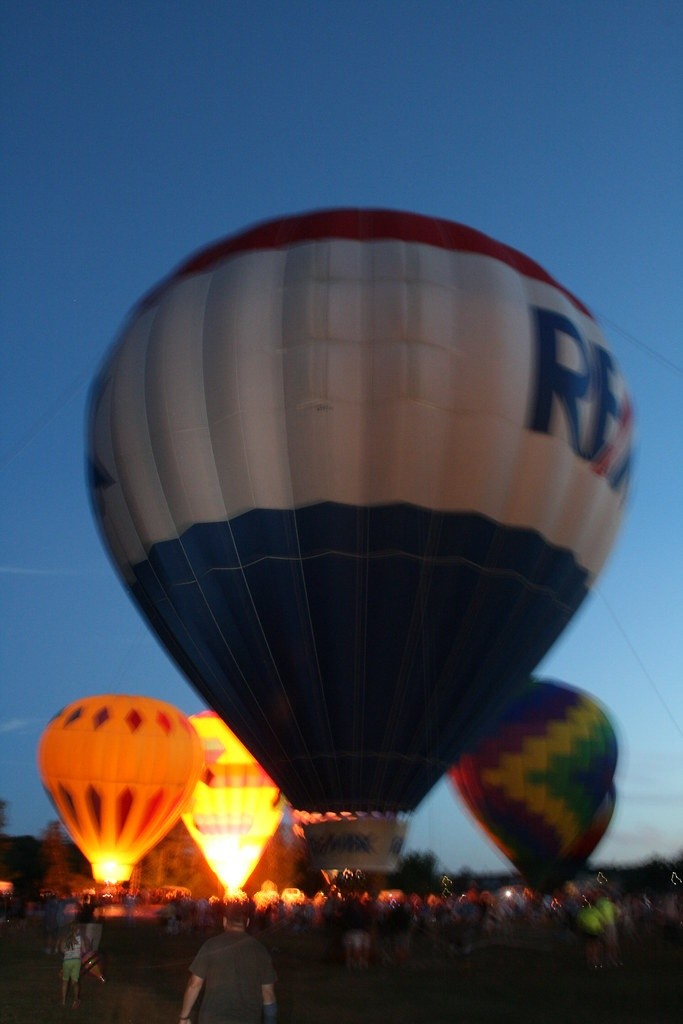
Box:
[39,693,205,905]
[450,678,619,890]
[180,708,285,903]
[84,206,638,923]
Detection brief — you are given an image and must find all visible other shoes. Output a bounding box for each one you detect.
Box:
[59,1001,65,1009]
[71,1000,82,1009]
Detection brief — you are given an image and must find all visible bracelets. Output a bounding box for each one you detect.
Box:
[179,1017,189,1020]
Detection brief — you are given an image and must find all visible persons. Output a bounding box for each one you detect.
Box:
[54,888,76,955]
[179,900,278,1024]
[45,895,64,955]
[167,881,683,968]
[124,892,138,928]
[60,921,86,1009]
